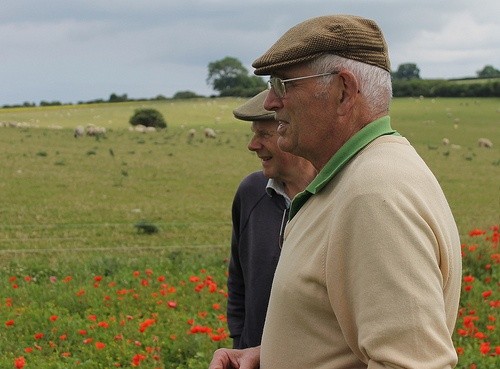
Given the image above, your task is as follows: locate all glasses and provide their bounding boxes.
[267,70,361,98]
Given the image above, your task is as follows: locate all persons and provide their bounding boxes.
[224,85,322,352]
[208,13,463,369]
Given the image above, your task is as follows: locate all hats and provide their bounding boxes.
[252,15,390,76]
[232,89,277,121]
